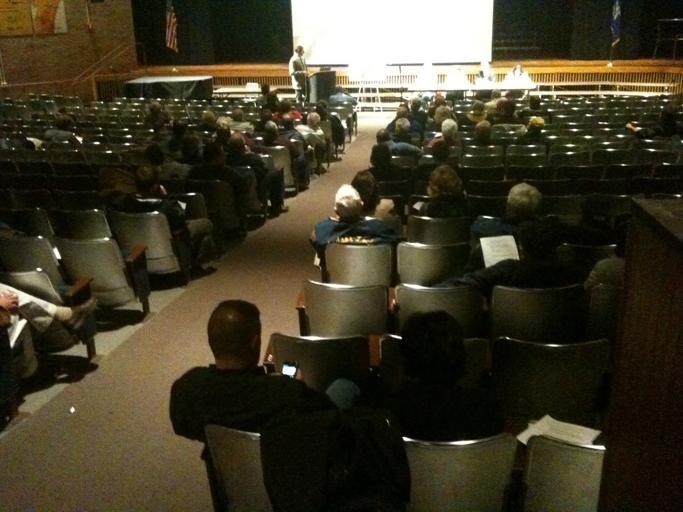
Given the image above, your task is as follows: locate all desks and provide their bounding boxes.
[125,76,213,99]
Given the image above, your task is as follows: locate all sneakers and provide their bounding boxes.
[62,296,96,334]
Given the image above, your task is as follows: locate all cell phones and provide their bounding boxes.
[281,360,299,379]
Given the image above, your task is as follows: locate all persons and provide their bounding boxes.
[0,46,683,511]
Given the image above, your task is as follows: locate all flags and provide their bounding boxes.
[609,0,621,49]
[164,2,180,53]
